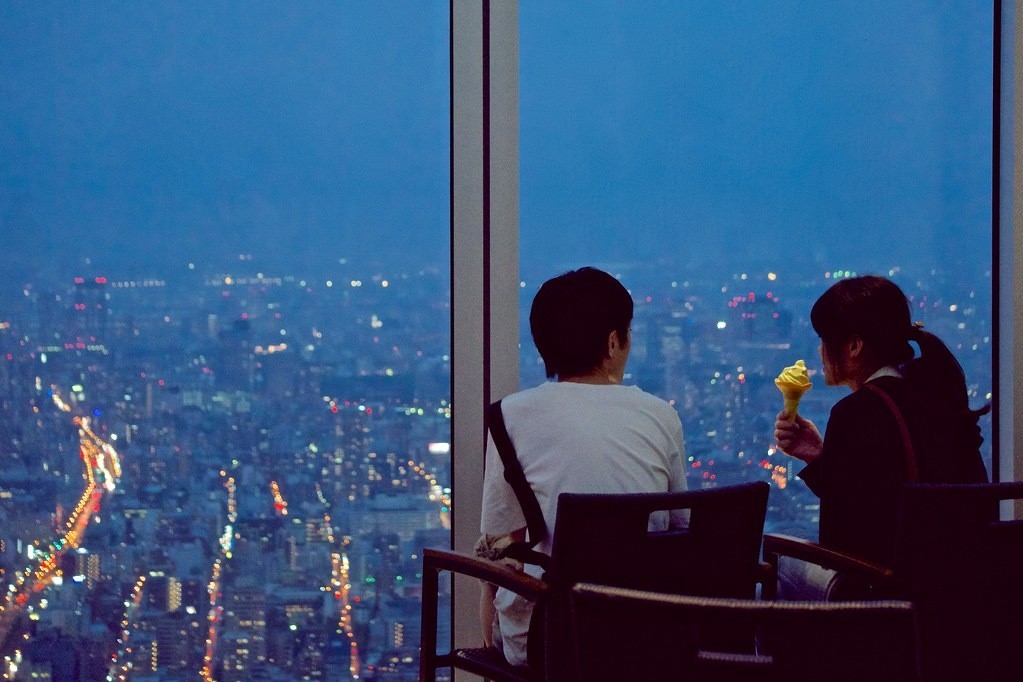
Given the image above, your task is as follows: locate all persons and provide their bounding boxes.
[479,267,693,668]
[756,276,999,612]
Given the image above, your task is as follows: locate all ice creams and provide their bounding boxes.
[774,360,813,423]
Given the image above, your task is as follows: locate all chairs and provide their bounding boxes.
[763,481,1022,682]
[567,584,923,682]
[420,481,770,682]
